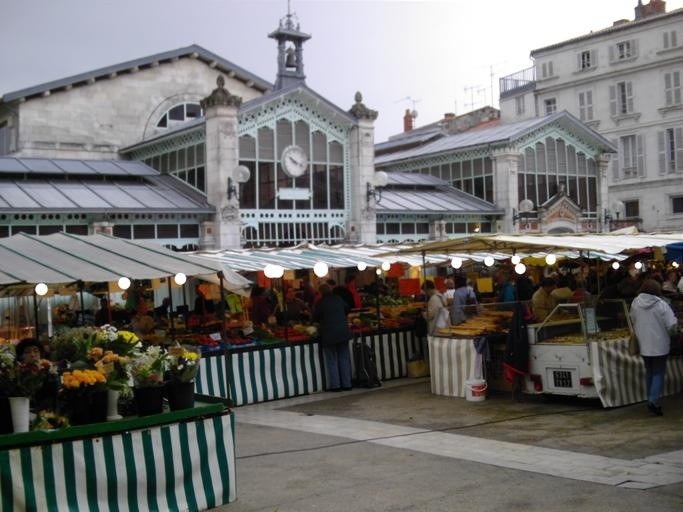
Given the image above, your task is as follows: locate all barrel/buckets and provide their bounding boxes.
[465,379,487,401]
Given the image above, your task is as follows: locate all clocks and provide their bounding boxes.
[282,146,309,177]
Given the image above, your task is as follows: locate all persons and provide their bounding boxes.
[276,286,313,326]
[93,297,119,326]
[416,279,448,341]
[311,283,353,394]
[662,271,679,293]
[567,268,583,288]
[529,275,558,323]
[13,336,50,366]
[151,296,170,321]
[628,279,679,416]
[191,284,217,313]
[451,274,481,325]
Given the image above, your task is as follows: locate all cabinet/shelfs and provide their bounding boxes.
[1,391,237,508]
[429,318,683,405]
[173,321,431,406]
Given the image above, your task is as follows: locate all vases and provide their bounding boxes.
[9,396,31,434]
[166,382,195,412]
[107,389,123,420]
[133,385,166,417]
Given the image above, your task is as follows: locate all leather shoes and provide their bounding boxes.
[647,401,664,417]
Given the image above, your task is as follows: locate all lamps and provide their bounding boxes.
[365,171,388,205]
[513,264,526,276]
[263,265,283,279]
[33,283,48,296]
[511,255,520,264]
[545,253,556,265]
[512,199,538,225]
[314,262,328,278]
[484,256,494,267]
[174,273,186,287]
[118,277,131,291]
[381,261,390,273]
[377,268,381,275]
[603,201,625,225]
[226,165,250,203]
[612,262,620,270]
[358,262,366,271]
[451,256,463,269]
[634,261,643,269]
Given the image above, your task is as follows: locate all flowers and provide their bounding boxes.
[35,411,67,429]
[0,346,60,395]
[163,347,200,384]
[132,344,165,385]
[48,324,142,389]
